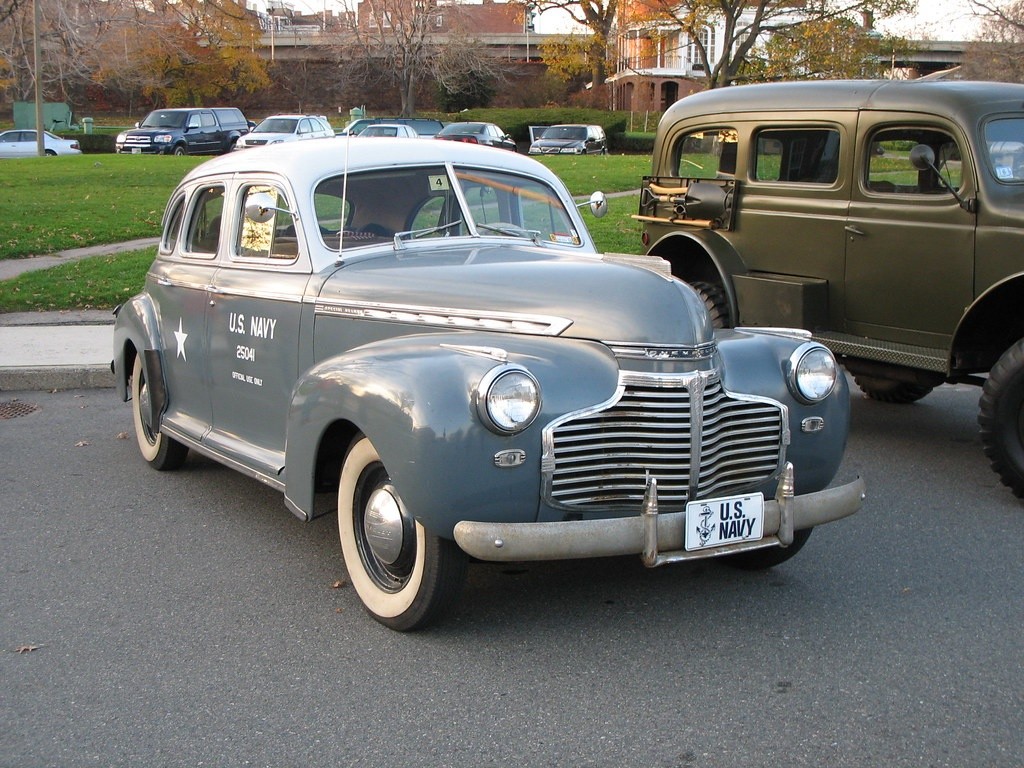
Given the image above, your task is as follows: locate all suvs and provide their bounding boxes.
[337,117,445,138]
[234,114,334,152]
[632,79,1024,495]
[113,107,251,157]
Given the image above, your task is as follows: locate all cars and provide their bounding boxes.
[0,130,83,159]
[435,121,522,153]
[529,124,608,157]
[356,124,417,138]
[108,135,865,630]
